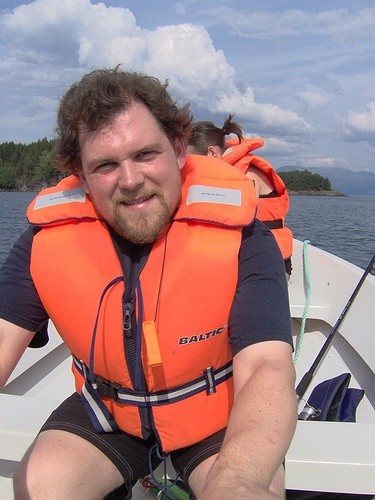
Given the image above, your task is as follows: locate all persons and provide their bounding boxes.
[0,66,296,500]
[184,121,293,284]
